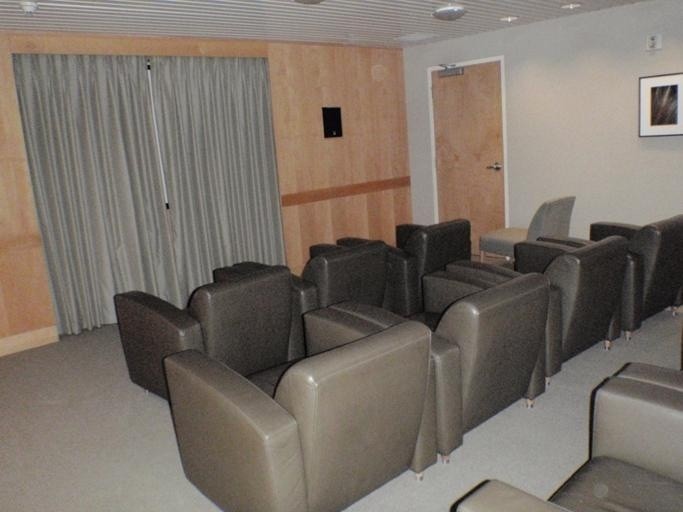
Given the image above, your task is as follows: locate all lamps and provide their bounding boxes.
[432,5,465,21]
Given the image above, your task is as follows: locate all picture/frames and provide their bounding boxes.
[637,72,682,137]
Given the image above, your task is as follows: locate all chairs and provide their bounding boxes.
[477,196,576,264]
[454,375,683,512]
[328,267,551,456]
[447,235,630,374]
[113,265,301,400]
[536,213,682,342]
[163,306,439,512]
[617,361,682,391]
[231,240,387,361]
[336,219,472,312]
